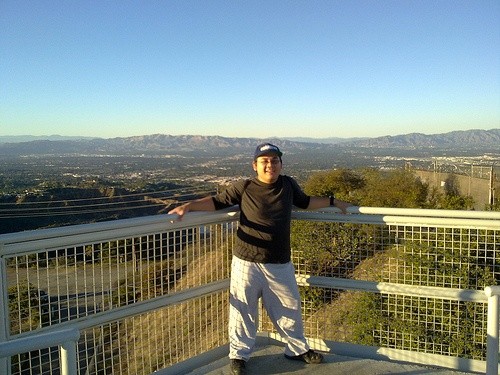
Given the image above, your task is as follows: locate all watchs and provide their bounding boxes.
[329,195,335,207]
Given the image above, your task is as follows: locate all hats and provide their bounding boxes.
[255,143,282,160]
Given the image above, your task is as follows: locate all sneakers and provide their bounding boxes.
[284,349,322,363]
[230,359,247,375]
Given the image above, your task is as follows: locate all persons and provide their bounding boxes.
[167,142,354,375]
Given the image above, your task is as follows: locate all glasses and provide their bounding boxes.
[257,160,281,164]
